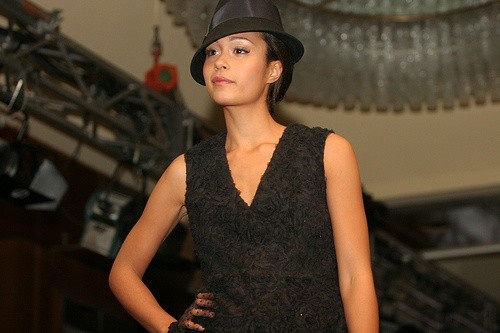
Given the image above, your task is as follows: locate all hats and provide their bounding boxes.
[189,0,304,86]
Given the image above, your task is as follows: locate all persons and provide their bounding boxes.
[108,0,380,333]
